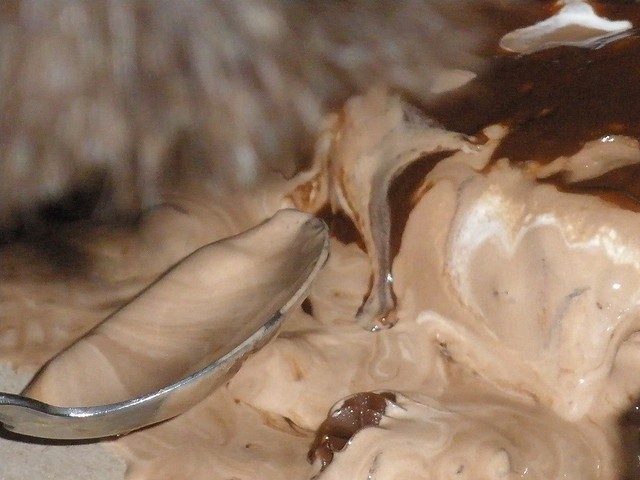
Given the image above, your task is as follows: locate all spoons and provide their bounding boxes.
[0,209,332,441]
